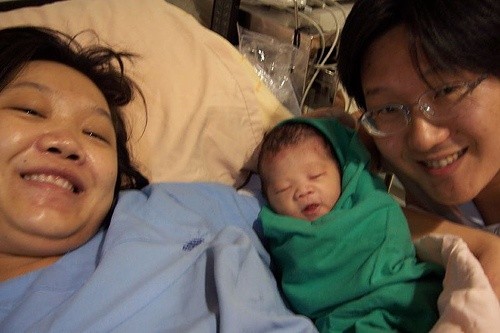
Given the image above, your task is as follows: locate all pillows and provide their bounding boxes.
[0,0,296,194]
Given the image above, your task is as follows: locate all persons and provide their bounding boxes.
[250,113,445,333]
[0,23,321,333]
[337,1,500,237]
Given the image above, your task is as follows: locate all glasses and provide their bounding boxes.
[357,71,496,145]
